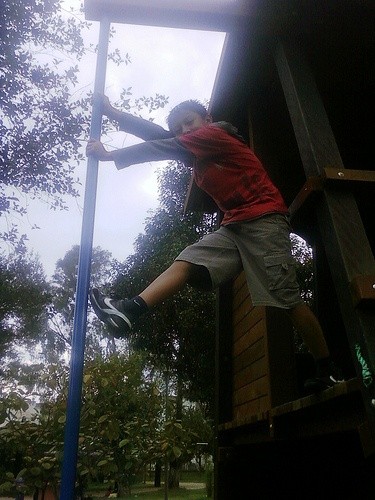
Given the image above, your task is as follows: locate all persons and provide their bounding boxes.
[86,94,346,388]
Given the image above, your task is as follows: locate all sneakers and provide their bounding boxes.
[89,288,135,339]
[304,369,341,392]
